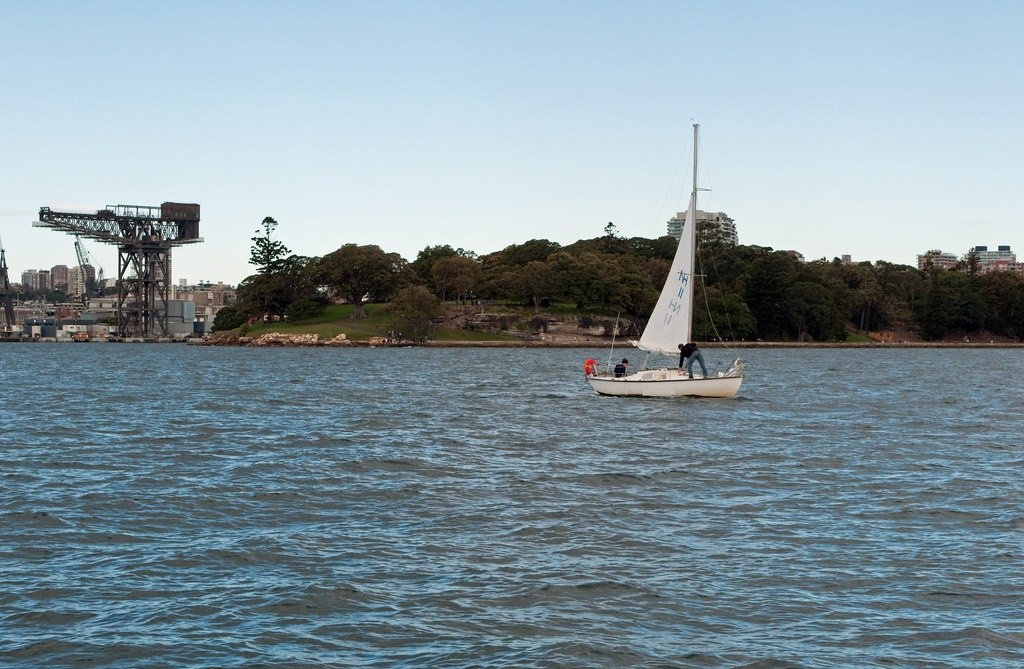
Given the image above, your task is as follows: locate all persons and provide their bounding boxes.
[614,358,628,378]
[385,328,429,345]
[678,343,708,379]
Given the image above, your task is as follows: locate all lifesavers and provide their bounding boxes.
[585,359,596,375]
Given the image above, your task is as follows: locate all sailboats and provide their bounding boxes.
[584,121,746,399]
